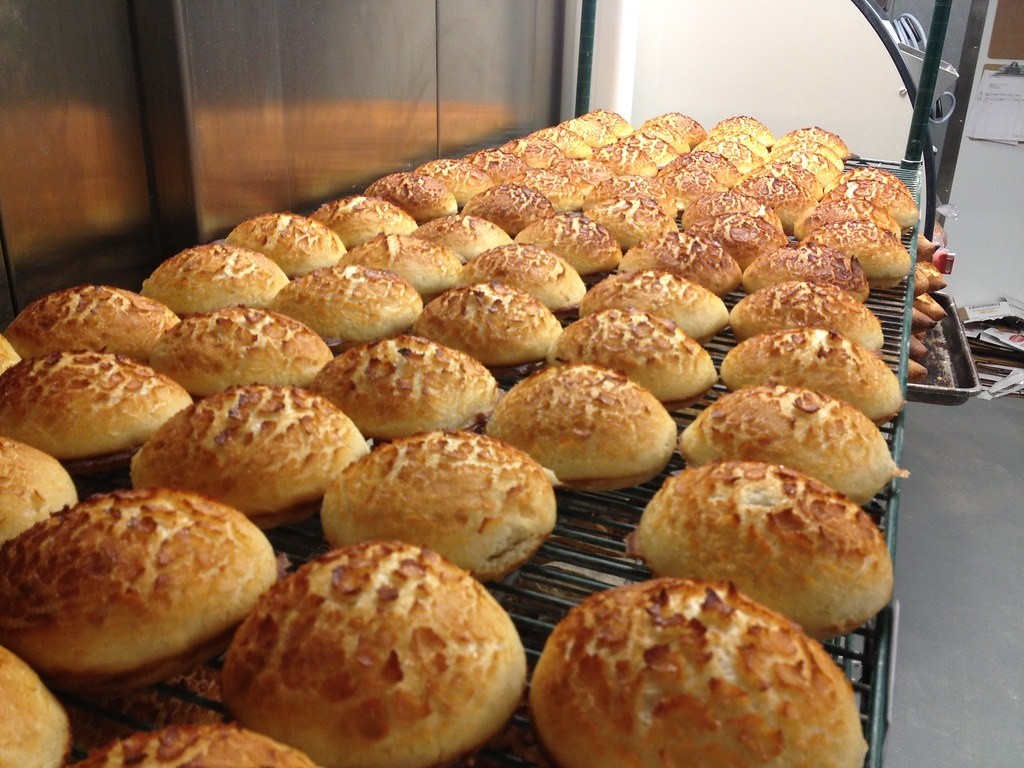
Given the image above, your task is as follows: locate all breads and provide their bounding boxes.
[0,110,956,768]
